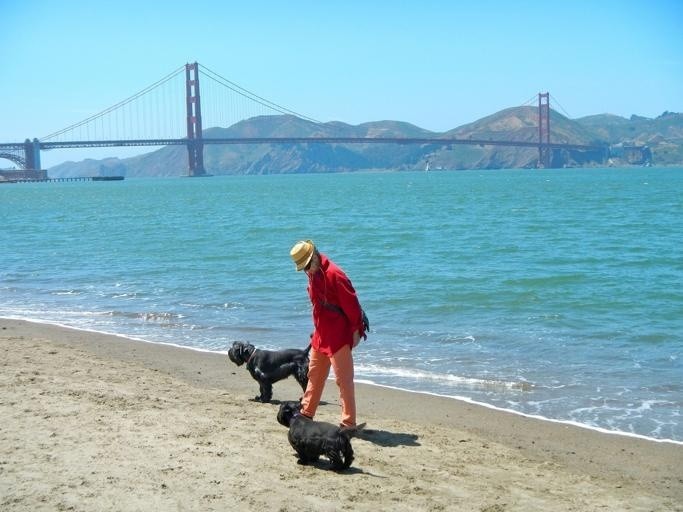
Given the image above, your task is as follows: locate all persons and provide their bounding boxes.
[289,238,367,436]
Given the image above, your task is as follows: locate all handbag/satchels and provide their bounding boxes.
[360,309,369,340]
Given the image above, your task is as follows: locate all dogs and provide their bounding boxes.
[227,333,314,403]
[276,401,367,472]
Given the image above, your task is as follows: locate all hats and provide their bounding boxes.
[291,240,314,270]
